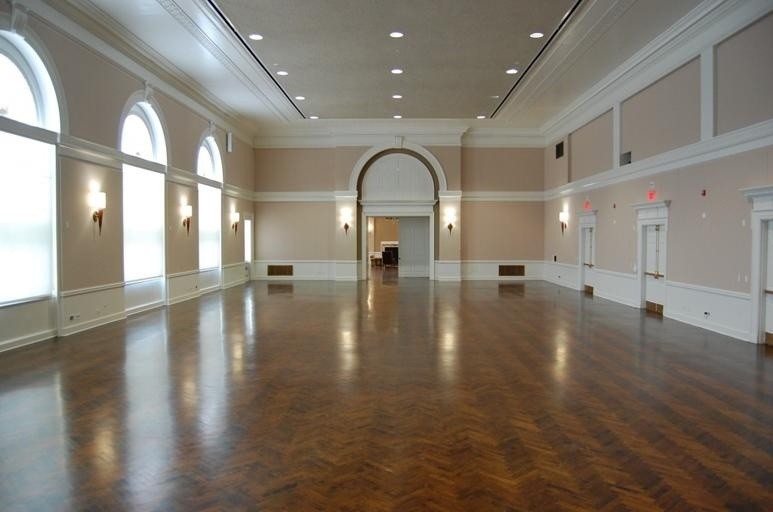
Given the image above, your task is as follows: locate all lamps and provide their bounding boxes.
[183,206,192,229]
[93,192,106,228]
[559,212,567,229]
[232,213,239,229]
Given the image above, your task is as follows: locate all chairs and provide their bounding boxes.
[382,251,396,271]
